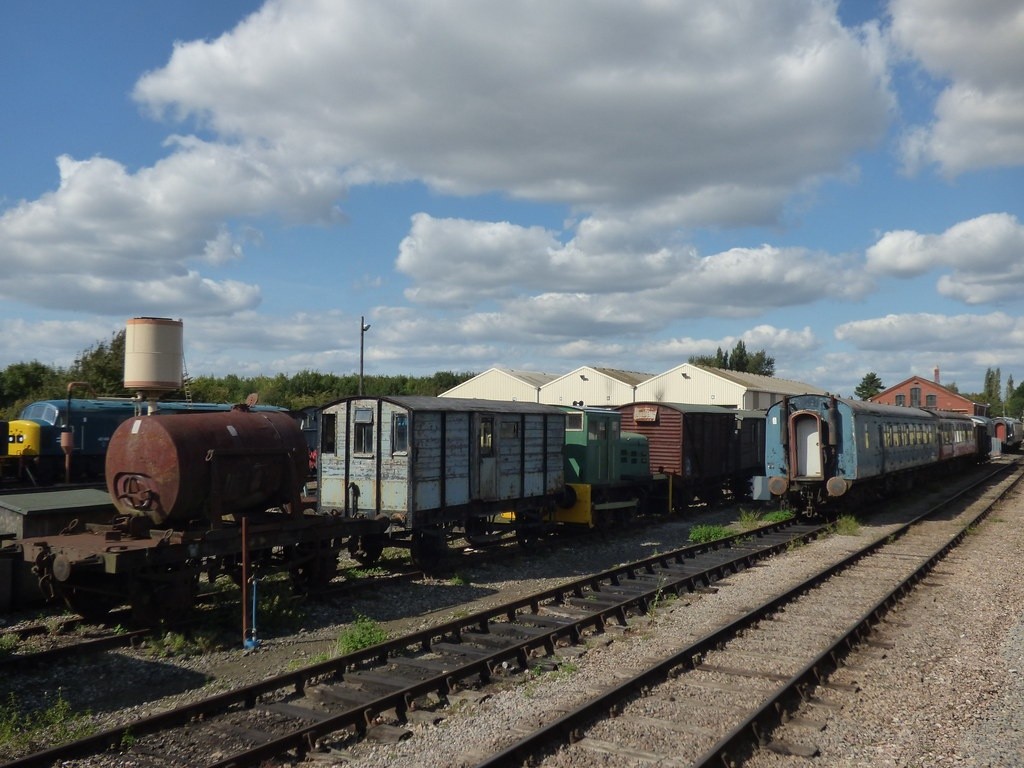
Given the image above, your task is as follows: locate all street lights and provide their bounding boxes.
[359,315,371,397]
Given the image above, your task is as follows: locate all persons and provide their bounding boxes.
[308,447,317,471]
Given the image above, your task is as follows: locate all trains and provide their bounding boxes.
[1,395,768,620]
[765,392,1024,519]
